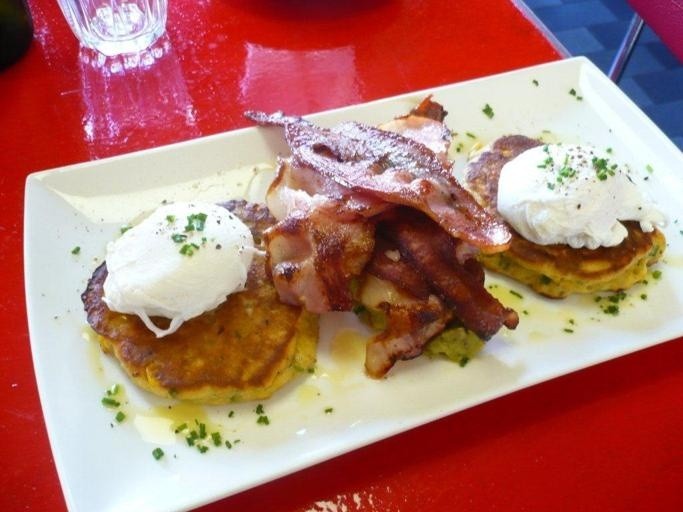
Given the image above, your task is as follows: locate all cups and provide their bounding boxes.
[77,34,203,163]
[54,0,169,57]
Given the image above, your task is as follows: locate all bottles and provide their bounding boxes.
[0,0,34,71]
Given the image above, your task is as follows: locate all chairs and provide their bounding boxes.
[608,0,683,85]
